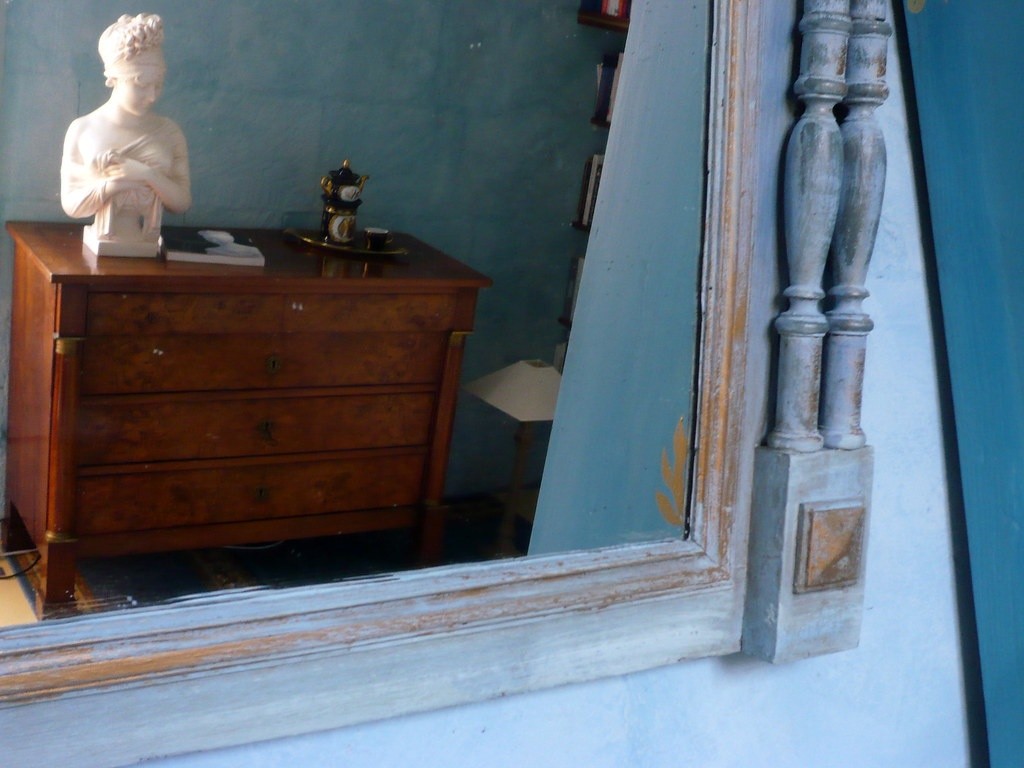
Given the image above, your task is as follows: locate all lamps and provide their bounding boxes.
[465,353,564,559]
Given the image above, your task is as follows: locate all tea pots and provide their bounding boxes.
[321,159,370,202]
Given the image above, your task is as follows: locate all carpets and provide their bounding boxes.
[7,498,540,620]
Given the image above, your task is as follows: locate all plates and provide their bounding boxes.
[304,230,409,254]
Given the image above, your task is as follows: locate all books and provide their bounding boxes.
[577,155,605,227]
[596,51,624,123]
[161,231,264,267]
[580,0,628,19]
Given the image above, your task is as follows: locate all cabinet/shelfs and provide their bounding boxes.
[560,12,633,332]
[0,219,495,620]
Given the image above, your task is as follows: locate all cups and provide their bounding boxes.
[364,226,394,250]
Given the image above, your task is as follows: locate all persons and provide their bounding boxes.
[60,12,193,242]
